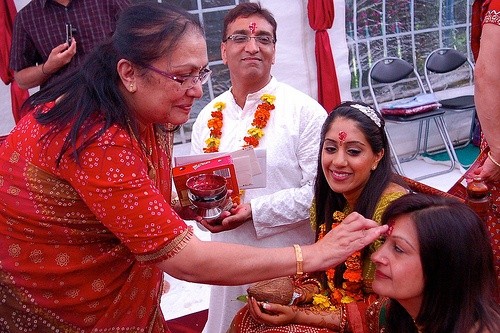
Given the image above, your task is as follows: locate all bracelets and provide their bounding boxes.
[488,151,500,167]
[41,64,50,76]
[294,244,303,278]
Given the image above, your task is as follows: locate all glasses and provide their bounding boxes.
[223,34,277,46]
[145,64,213,90]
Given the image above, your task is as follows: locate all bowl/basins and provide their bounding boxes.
[185,173,227,198]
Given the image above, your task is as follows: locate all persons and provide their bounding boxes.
[369,194,500,333]
[469,0,487,147]
[226,100,408,333]
[9,0,158,100]
[0,1,389,333]
[190,1,330,333]
[466,0,500,314]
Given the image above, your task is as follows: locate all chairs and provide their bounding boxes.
[368,56,456,182]
[421,48,484,175]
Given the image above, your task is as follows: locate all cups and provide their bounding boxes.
[187,190,227,218]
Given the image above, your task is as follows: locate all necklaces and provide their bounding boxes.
[202,93,276,153]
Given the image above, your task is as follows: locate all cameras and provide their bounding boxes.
[65,23,77,48]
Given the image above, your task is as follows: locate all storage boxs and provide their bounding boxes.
[172,155,240,208]
